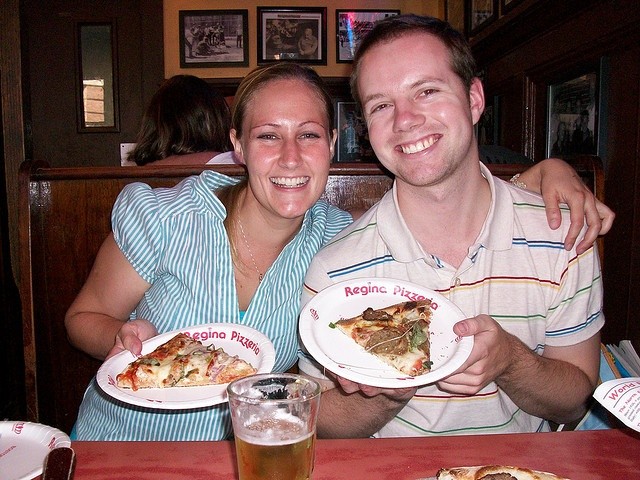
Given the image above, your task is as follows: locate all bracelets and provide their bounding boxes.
[509,172,527,191]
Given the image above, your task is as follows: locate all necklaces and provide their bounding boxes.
[236,186,265,283]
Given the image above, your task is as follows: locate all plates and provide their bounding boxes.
[95,321,277,411]
[298,277,476,390]
[0,417,74,480]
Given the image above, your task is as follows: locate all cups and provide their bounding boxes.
[223,371,322,480]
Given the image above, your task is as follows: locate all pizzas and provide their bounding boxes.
[335,298,435,377]
[116,332,258,392]
[438,466,560,480]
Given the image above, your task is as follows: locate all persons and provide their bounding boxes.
[185,22,232,59]
[340,35,344,47]
[267,20,318,59]
[236,24,243,47]
[283,14,605,439]
[126,76,232,165]
[548,109,595,159]
[64,61,616,442]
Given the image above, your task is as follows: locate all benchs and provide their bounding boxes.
[11,161,620,434]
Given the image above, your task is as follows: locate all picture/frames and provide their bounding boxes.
[178,8,250,68]
[256,5,328,65]
[524,74,609,162]
[335,7,402,63]
[331,93,384,163]
